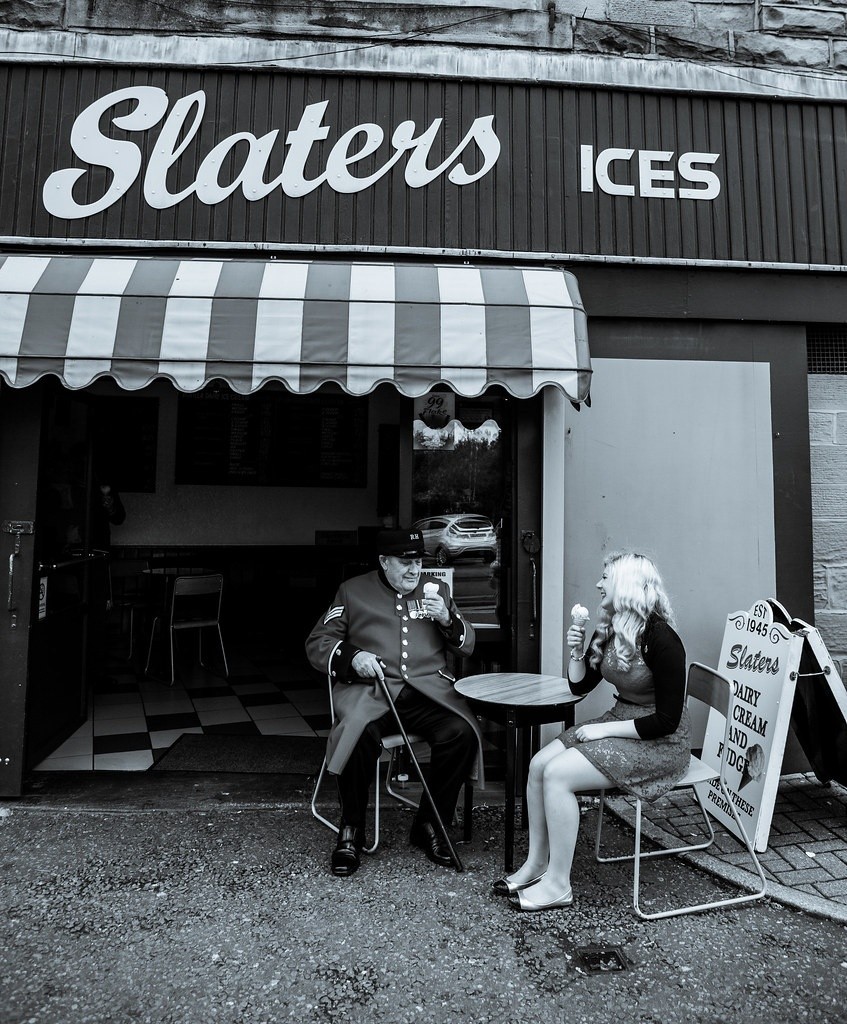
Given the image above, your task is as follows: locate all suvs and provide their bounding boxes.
[412,513,497,567]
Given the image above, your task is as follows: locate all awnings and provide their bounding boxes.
[0,248,594,413]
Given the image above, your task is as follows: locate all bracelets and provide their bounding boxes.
[570,649,585,662]
[353,650,363,658]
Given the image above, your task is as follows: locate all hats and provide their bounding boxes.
[379,530,433,558]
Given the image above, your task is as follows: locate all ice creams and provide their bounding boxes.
[100,485,111,496]
[571,603,590,632]
[423,582,439,621]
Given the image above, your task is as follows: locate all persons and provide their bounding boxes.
[62,442,126,547]
[493,548,691,910]
[305,528,486,878]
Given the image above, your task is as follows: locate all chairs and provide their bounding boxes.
[594,661,768,920]
[312,675,457,854]
[144,574,229,686]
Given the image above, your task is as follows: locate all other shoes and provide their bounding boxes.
[491,871,548,894]
[506,887,573,910]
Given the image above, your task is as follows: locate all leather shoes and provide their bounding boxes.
[332,823,366,876]
[409,819,455,868]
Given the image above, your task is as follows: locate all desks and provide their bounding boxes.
[453,672,590,872]
